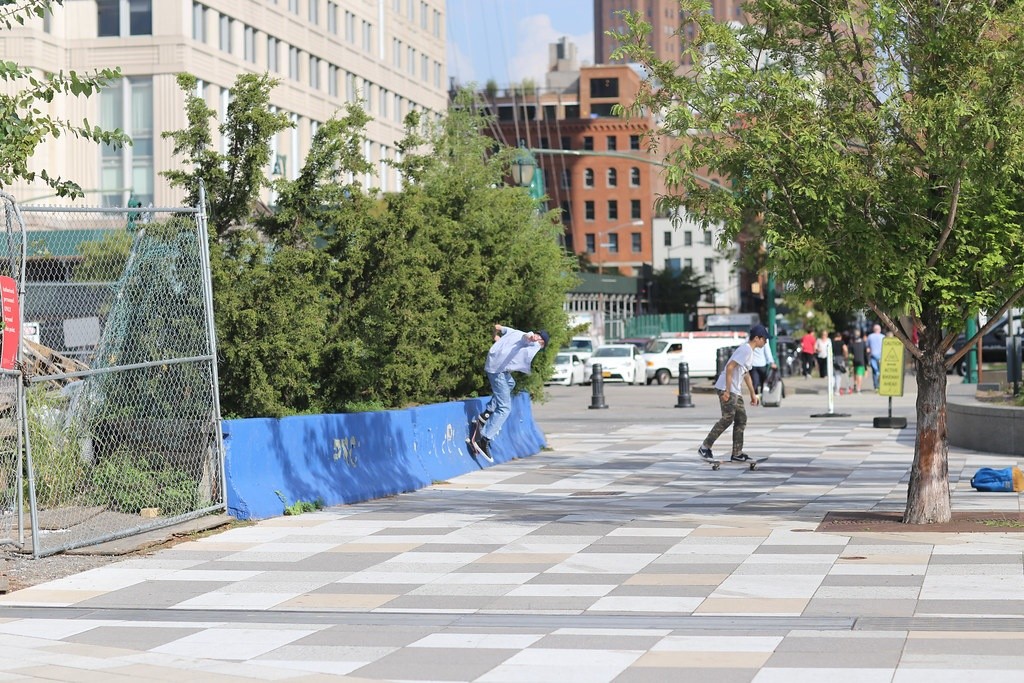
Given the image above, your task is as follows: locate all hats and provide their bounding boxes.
[750,325,773,339]
[536,330,548,348]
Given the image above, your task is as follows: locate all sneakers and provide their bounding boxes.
[731,452,756,463]
[478,409,491,424]
[698,445,714,462]
[472,437,494,463]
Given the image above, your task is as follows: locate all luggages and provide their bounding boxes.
[761,365,782,406]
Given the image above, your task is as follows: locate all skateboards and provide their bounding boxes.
[699,454,769,471]
[465,414,482,455]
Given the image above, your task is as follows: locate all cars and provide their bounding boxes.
[585,344,649,386]
[542,352,584,386]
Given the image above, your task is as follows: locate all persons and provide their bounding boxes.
[732,324,899,407]
[697,324,775,464]
[465,323,551,462]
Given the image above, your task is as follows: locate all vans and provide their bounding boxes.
[641,337,748,387]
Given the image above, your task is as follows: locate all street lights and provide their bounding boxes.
[510,139,538,187]
